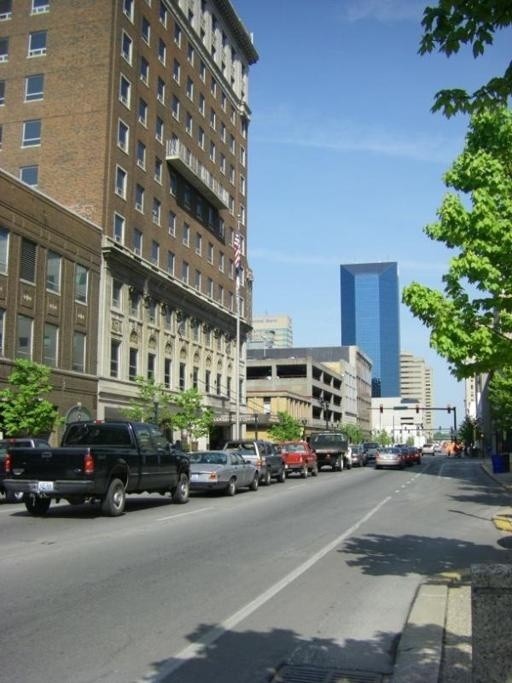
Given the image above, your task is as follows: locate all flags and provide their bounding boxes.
[233,220,242,268]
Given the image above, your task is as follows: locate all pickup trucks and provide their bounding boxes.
[0,436,52,502]
[3,419,192,517]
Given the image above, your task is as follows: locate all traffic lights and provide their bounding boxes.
[416,404,419,412]
[448,405,451,413]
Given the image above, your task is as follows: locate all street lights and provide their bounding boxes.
[253,409,259,440]
[480,433,485,457]
[380,403,384,412]
[151,392,160,418]
[301,416,308,440]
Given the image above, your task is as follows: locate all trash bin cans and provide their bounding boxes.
[492,454,510,473]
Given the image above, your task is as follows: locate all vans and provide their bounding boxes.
[222,439,286,484]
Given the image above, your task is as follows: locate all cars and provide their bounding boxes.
[185,450,261,494]
[275,440,320,477]
[349,439,443,470]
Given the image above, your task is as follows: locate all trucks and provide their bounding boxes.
[309,429,353,471]
